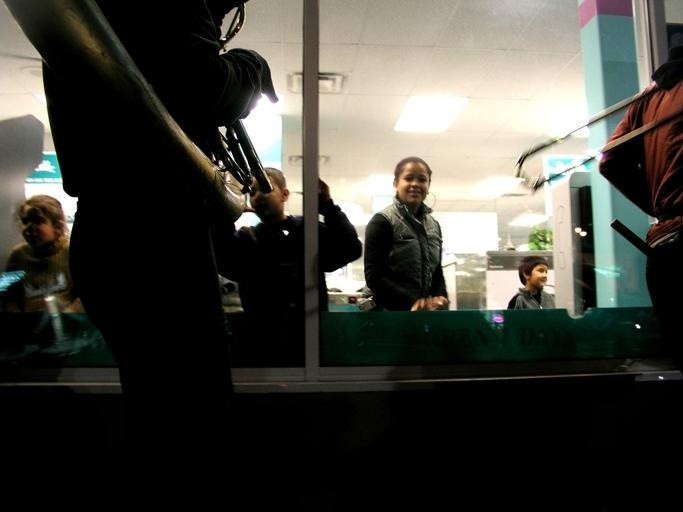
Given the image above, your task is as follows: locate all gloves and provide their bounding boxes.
[235,49,278,103]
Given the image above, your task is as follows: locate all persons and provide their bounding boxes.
[362,157,452,312]
[211,166,363,313]
[597,43,682,382]
[39,2,279,512]
[1,193,88,315]
[507,254,557,311]
